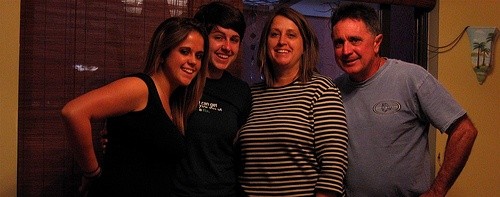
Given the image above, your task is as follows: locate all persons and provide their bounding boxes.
[231,6,348,197]
[99,1,253,196]
[323,2,478,197]
[60,16,210,197]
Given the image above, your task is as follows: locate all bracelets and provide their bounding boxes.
[80,163,103,180]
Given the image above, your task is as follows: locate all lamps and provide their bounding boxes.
[466,26,500,85]
